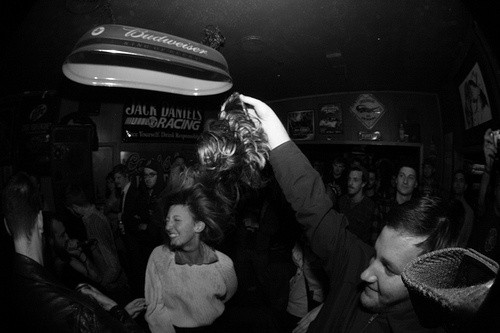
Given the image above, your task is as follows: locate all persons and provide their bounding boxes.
[239,92,468,333]
[144,192,238,333]
[0,129,500,333]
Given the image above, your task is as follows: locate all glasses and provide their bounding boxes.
[143,173,159,178]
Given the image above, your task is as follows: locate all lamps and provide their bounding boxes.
[60,24,234,97]
